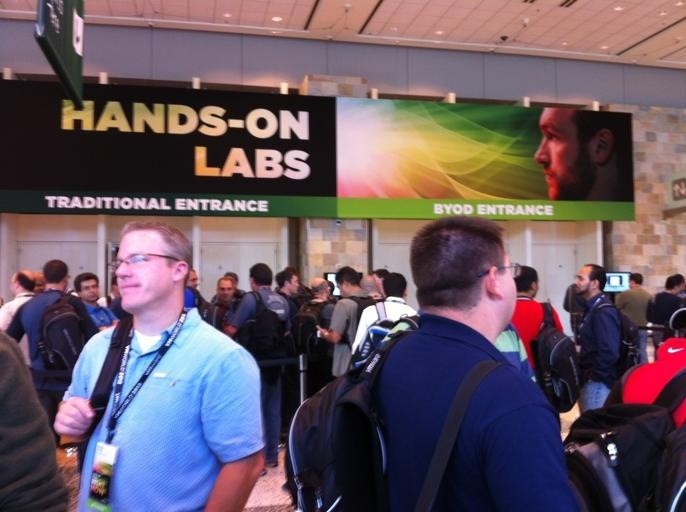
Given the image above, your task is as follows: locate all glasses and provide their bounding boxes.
[478,264,523,278]
[107,253,181,272]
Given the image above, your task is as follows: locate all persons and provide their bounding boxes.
[534,108,631,200]
[602,307,686,511]
[52,219,266,510]
[1,260,129,471]
[183,262,418,466]
[493,263,686,427]
[353,216,590,509]
[0,329,73,511]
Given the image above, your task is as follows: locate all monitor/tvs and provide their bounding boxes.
[324,273,362,297]
[603,272,631,293]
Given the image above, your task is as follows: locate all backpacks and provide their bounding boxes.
[562,362,686,512]
[528,302,584,412]
[236,291,283,357]
[36,294,90,371]
[292,300,336,353]
[594,302,641,378]
[283,329,390,512]
[351,302,395,369]
